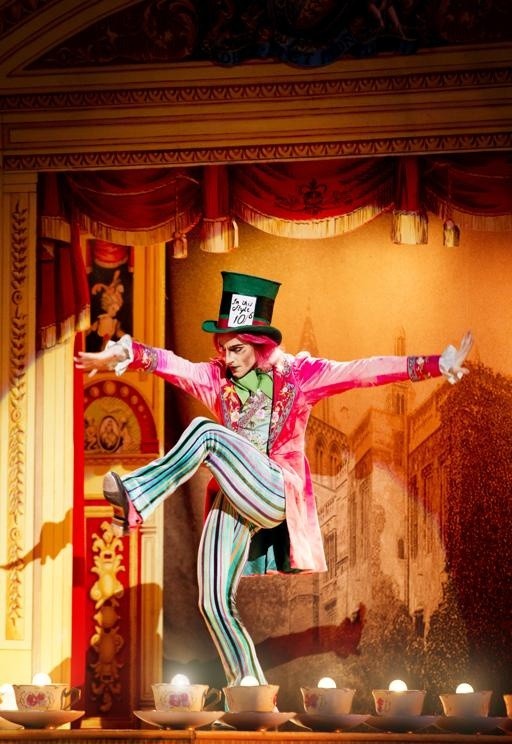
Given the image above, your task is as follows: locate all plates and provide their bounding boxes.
[290,714,373,735]
[1,710,86,730]
[435,716,502,734]
[133,710,226,731]
[218,712,297,731]
[365,715,438,733]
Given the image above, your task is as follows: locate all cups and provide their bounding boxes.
[9,682,80,710]
[150,682,221,711]
[298,685,356,710]
[436,689,492,710]
[370,686,427,708]
[221,685,279,710]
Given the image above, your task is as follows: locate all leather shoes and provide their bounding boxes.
[102,470,143,539]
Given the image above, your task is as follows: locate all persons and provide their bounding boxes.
[74,333,474,730]
[87,292,125,352]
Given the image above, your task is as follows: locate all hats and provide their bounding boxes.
[201,270,283,347]
